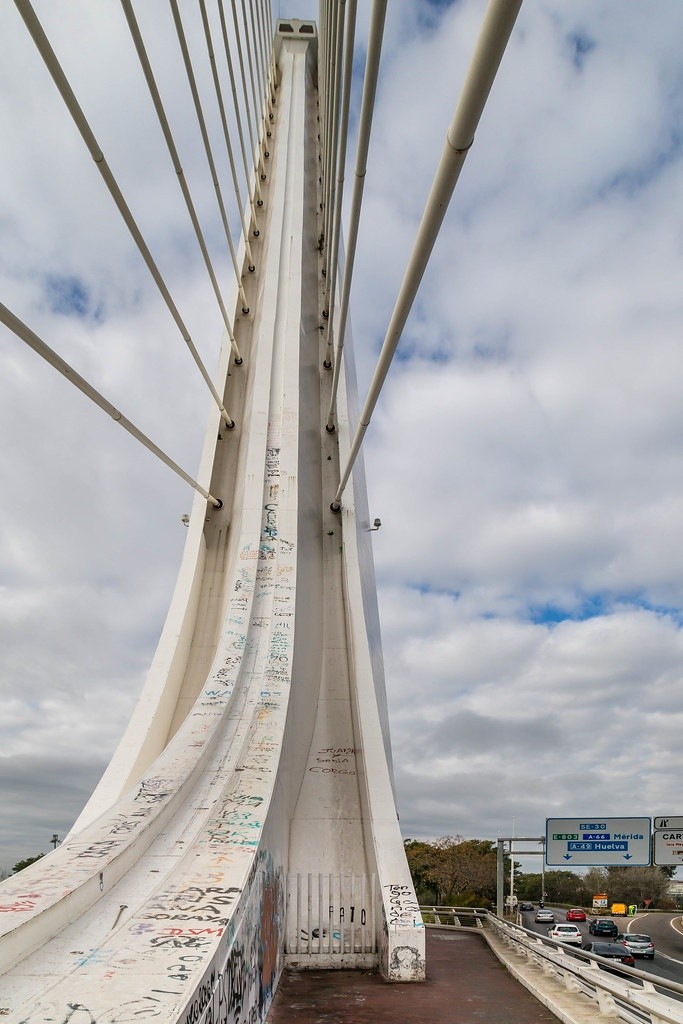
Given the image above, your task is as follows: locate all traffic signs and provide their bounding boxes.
[545,816,652,867]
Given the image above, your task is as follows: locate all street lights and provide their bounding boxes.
[509,815,516,916]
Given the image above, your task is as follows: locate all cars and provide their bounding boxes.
[519,902,535,912]
[534,909,555,924]
[574,941,636,978]
[546,923,583,947]
[565,908,587,922]
[611,933,655,960]
[588,918,618,937]
[610,902,628,917]
